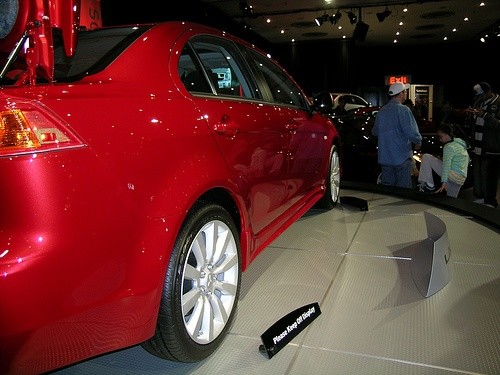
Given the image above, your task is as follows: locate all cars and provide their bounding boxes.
[346,105,473,185]
[309,91,373,114]
[0,19,344,375]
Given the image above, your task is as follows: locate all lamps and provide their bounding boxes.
[329,9,342,25]
[315,10,329,27]
[347,8,356,24]
[376,4,391,22]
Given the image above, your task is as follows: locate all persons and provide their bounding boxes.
[402,98,418,127]
[333,97,347,113]
[466,82,500,208]
[415,123,471,198]
[421,97,428,120]
[372,81,422,188]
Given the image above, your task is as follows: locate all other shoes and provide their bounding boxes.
[416,182,435,192]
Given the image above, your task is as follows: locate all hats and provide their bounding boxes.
[474,82,491,96]
[388,81,411,96]
